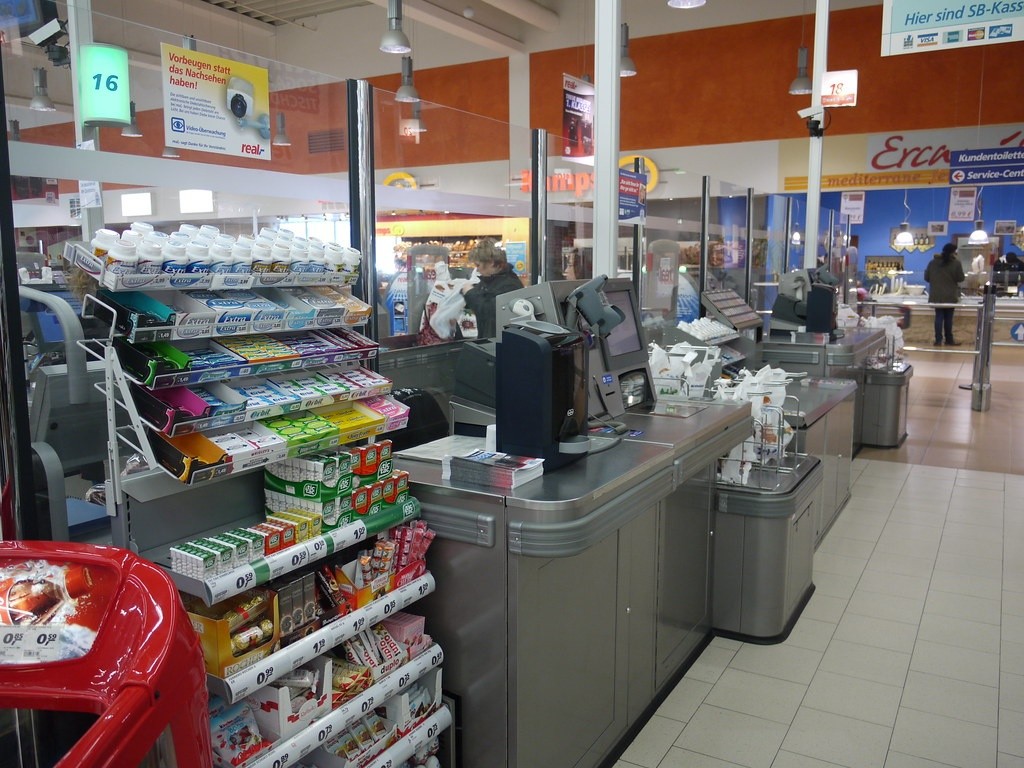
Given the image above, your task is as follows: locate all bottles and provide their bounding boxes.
[1,566,93,625]
[91,221,362,274]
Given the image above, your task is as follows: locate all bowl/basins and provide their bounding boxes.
[905,285,926,295]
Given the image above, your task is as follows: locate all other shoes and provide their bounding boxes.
[945,339,961,346]
[934,341,941,346]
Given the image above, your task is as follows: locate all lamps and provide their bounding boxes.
[379,0,411,54]
[396,57,421,103]
[894,188,914,246]
[793,199,800,241]
[619,22,638,78]
[789,48,813,94]
[968,187,988,244]
[410,103,427,132]
[7,69,291,159]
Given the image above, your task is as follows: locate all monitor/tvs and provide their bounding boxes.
[0,0,59,43]
[605,290,643,356]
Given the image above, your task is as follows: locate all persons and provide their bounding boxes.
[454,236,525,341]
[564,246,592,279]
[925,243,965,345]
[994,251,1024,287]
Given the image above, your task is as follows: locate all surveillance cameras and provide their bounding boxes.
[226,75,255,118]
[797,105,823,119]
[27,18,66,47]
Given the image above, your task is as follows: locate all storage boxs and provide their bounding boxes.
[60,239,442,768]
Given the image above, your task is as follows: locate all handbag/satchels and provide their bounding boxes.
[415,279,472,344]
